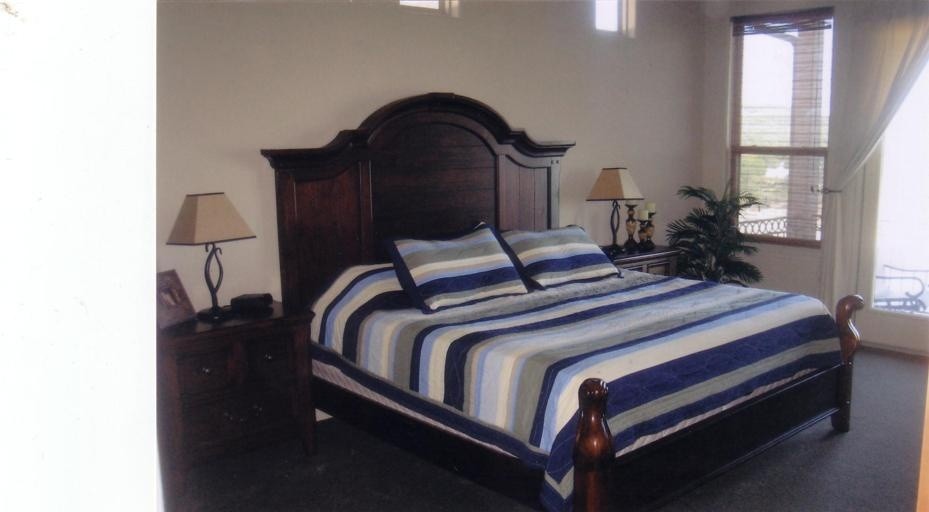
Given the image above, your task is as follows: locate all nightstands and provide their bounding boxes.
[157,299,318,496]
[599,245,682,276]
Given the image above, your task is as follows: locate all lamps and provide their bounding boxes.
[165,192,256,320]
[585,168,645,257]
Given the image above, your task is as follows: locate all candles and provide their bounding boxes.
[638,209,649,220]
[625,200,637,205]
[645,203,655,213]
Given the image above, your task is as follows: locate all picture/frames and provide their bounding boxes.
[156,270,198,332]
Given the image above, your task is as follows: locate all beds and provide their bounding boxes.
[262,92,865,512]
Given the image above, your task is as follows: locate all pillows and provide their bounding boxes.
[498,225,624,290]
[382,219,534,312]
